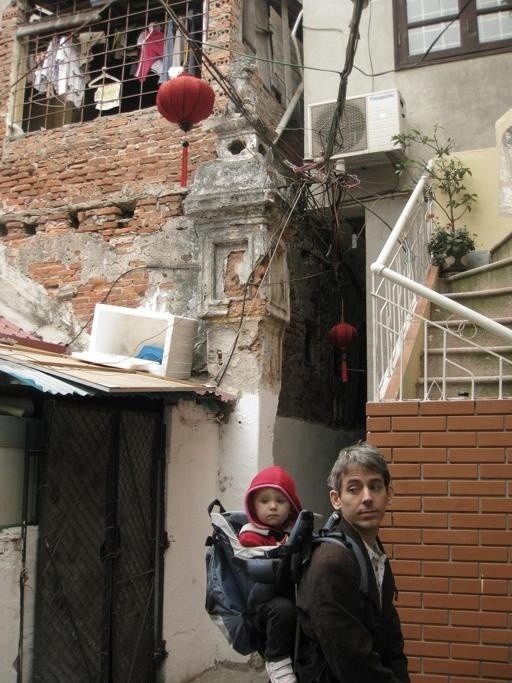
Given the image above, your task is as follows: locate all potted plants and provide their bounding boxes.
[387,123,480,276]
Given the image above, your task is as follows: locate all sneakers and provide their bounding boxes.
[265,656,297,683]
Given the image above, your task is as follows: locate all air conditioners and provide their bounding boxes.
[306,88,407,174]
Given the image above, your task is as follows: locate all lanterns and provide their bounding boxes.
[156,72,215,187]
[326,319,359,384]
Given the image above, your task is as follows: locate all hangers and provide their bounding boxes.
[88,62,120,86]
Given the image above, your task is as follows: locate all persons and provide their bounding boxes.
[296,443,411,682]
[238,465,302,682]
